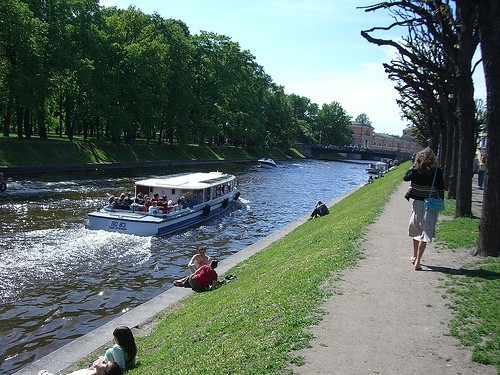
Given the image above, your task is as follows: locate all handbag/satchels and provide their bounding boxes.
[425,198,446,211]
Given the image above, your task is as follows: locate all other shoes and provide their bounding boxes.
[308,218,314,220]
[315,216,318,217]
[415,266,422,270]
[413,260,415,264]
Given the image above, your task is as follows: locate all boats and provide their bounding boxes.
[257,157,277,169]
[85,170,240,237]
[366,157,399,174]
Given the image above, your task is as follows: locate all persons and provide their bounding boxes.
[38,326,137,375]
[367,176,373,184]
[478,156,486,189]
[403,147,445,270]
[180,189,220,206]
[381,171,383,177]
[188,246,211,273]
[173,260,218,292]
[109,192,167,214]
[308,201,327,220]
